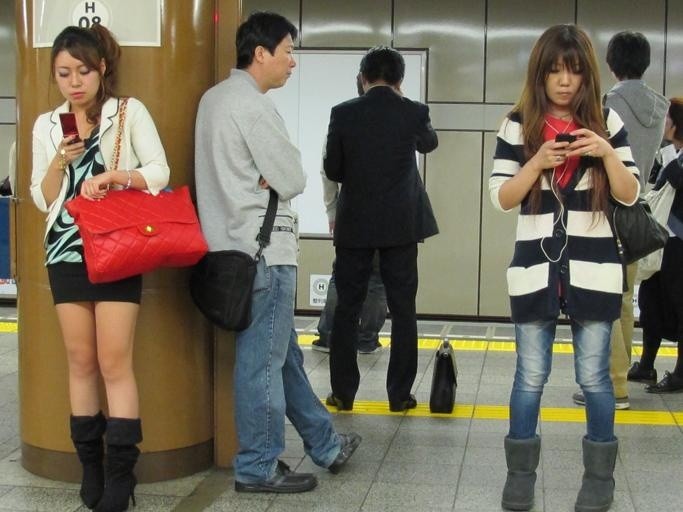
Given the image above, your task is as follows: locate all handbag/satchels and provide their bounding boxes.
[603,185,671,263]
[429,337,459,413]
[63,94,211,291]
[635,161,679,284]
[182,241,258,334]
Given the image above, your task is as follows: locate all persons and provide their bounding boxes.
[572,29,673,410]
[28,23,172,511]
[487,22,644,512]
[312,72,389,354]
[322,42,441,411]
[627,95,683,394]
[192,8,362,493]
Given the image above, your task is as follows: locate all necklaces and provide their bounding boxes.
[546,109,571,120]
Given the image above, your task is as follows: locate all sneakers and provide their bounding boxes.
[233,459,318,494]
[359,338,384,354]
[311,339,331,353]
[572,388,631,410]
[327,431,362,476]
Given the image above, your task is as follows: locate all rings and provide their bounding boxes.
[556,155,561,161]
[584,151,591,157]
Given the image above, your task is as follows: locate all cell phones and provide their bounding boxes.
[555,132,579,144]
[58,112,82,144]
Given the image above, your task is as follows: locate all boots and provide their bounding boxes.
[573,435,619,512]
[68,410,108,507]
[100,412,142,512]
[501,431,542,512]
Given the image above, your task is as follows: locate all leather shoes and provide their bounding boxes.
[628,360,658,384]
[387,392,417,413]
[326,391,355,411]
[644,369,683,394]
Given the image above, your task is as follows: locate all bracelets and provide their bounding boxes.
[124,168,133,190]
